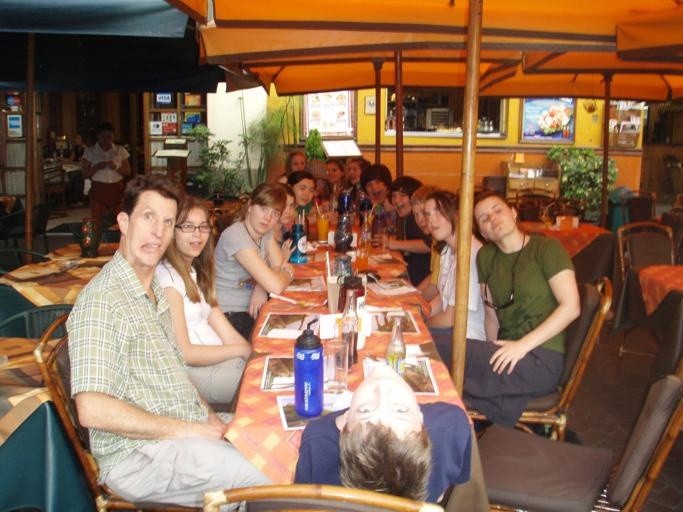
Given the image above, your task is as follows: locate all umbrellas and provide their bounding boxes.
[1,0,225,264]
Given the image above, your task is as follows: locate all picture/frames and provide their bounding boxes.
[298,86,358,145]
[517,92,579,146]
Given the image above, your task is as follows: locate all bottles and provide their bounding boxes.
[335,272,366,310]
[340,288,358,366]
[288,223,308,263]
[293,318,325,418]
[385,314,408,377]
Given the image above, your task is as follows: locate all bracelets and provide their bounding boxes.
[93,166,97,170]
[113,165,119,171]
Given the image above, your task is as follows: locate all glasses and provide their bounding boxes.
[484,271,515,309]
[176,225,214,233]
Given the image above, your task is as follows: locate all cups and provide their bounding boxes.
[315,182,388,271]
[322,338,350,391]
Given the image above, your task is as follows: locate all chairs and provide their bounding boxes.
[476,364,683,512]
[30,313,205,511]
[465,275,612,442]
[0,169,118,341]
[202,482,449,512]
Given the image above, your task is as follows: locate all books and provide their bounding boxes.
[1,91,76,196]
[149,91,206,183]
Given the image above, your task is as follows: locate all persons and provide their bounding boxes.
[295,369,471,511]
[72,133,88,159]
[64,174,273,510]
[82,122,131,217]
[210,150,583,430]
[149,196,253,405]
